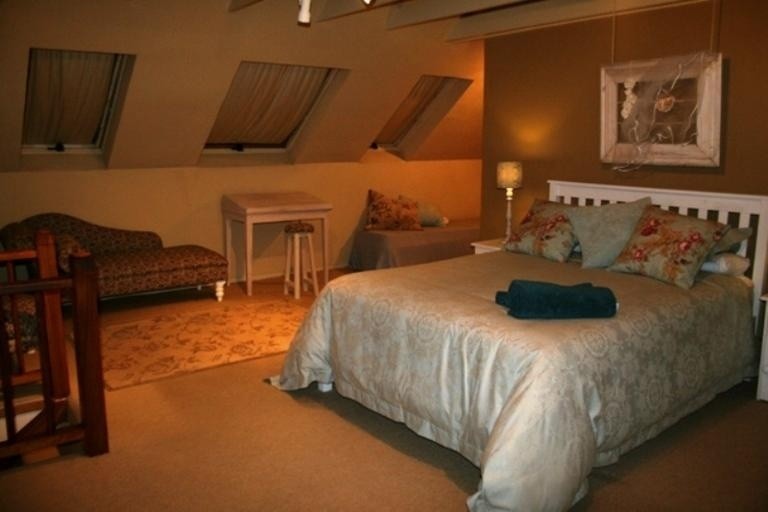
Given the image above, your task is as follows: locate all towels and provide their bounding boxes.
[494,281,592,307]
[507,277,615,322]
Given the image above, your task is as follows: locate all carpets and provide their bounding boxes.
[66,296,313,393]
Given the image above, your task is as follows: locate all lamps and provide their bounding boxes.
[494,159,524,246]
[296,0,318,26]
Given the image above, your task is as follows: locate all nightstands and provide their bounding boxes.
[470,236,511,256]
[751,295,768,402]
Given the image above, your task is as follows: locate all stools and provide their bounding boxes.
[279,219,320,300]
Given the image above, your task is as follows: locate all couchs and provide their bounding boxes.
[1,213,229,365]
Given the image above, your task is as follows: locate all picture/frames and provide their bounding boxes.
[597,55,722,168]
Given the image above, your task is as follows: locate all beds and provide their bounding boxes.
[260,177,767,511]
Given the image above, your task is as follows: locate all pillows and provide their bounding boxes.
[402,194,448,228]
[705,220,752,261]
[611,203,724,296]
[566,195,654,269]
[363,189,419,233]
[33,230,89,272]
[699,250,750,277]
[501,193,590,265]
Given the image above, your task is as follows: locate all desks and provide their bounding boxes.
[220,192,332,301]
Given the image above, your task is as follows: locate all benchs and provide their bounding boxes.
[349,219,479,276]
